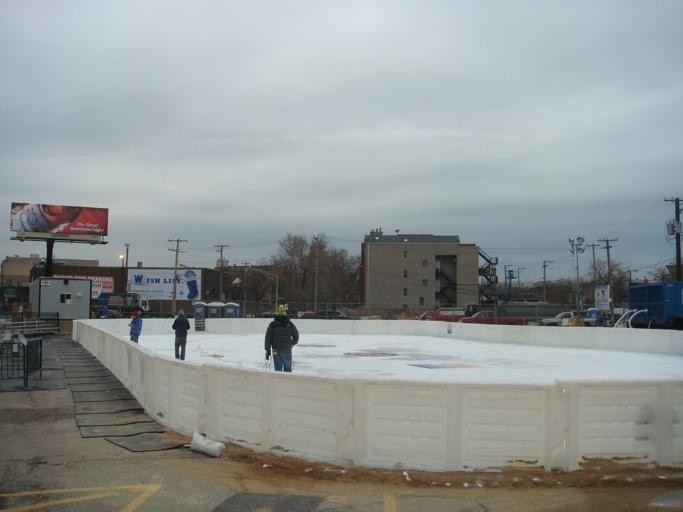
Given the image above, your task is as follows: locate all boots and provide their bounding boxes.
[180,355,185,360]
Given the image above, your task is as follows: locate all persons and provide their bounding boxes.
[128,309,142,343]
[172,309,190,360]
[265,304,299,372]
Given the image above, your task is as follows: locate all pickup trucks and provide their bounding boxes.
[540,312,596,327]
[410,308,525,326]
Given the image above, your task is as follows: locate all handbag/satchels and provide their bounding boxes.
[272,348,280,356]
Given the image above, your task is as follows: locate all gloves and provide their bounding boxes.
[128,322,135,328]
[265,350,271,360]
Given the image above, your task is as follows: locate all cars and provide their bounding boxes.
[302,309,360,320]
[258,308,298,318]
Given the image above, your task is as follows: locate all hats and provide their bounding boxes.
[179,309,184,315]
[277,305,287,315]
[131,310,140,315]
